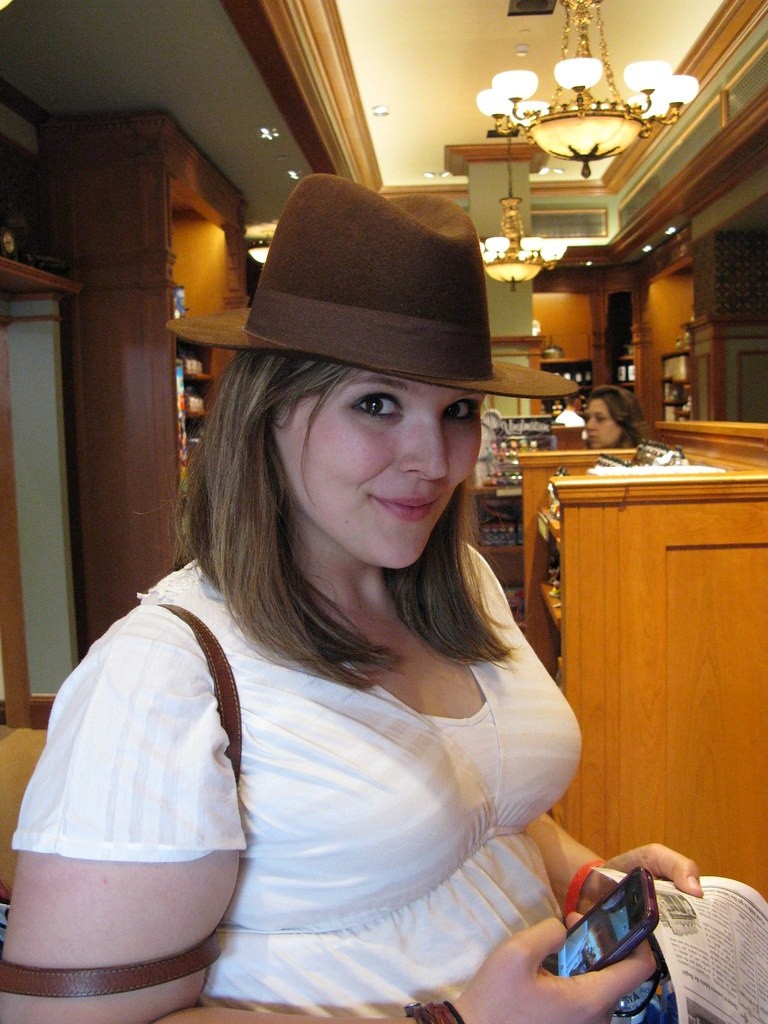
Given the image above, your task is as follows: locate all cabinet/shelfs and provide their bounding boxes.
[169,340,221,465]
[475,354,634,481]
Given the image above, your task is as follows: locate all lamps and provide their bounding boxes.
[474,127,570,291]
[473,0,704,180]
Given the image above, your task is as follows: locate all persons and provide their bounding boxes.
[0,173,707,1024]
[586,384,650,450]
[552,396,589,447]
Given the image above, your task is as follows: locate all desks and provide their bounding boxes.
[474,486,524,546]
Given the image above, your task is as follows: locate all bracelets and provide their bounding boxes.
[404,1001,466,1024]
[562,858,605,918]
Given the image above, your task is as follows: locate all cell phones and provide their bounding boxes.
[540,866,659,979]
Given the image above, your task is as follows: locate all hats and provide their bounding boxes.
[165,172,579,400]
